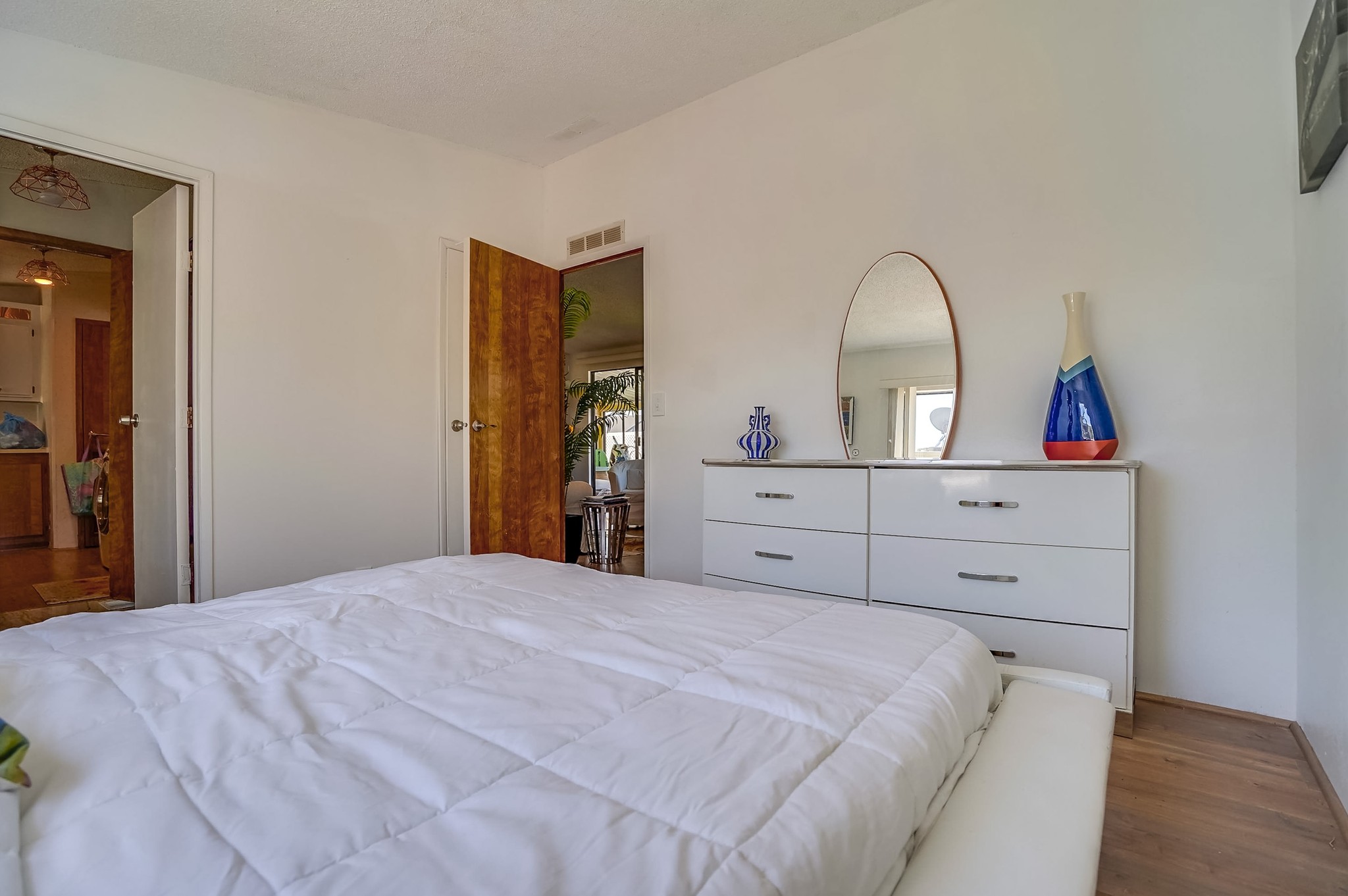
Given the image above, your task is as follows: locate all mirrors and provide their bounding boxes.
[837,252,959,458]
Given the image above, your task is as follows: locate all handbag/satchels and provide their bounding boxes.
[61,432,104,516]
[0,412,45,448]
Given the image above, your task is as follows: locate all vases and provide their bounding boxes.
[737,406,781,460]
[1042,292,1119,459]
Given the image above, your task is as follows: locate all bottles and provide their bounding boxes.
[736,407,780,459]
[1041,290,1120,462]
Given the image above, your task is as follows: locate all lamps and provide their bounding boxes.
[15,245,71,286]
[9,145,91,210]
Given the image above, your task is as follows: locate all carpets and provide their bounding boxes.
[31,573,110,605]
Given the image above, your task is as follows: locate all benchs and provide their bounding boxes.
[892,663,1114,896]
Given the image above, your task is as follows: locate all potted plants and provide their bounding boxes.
[559,288,644,564]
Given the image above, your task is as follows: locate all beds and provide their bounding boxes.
[0,551,1003,896]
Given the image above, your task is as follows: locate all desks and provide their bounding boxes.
[579,501,631,564]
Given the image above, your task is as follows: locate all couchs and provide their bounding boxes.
[609,459,644,531]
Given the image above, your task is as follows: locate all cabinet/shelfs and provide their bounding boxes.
[704,464,1140,738]
[0,453,49,549]
[0,318,43,402]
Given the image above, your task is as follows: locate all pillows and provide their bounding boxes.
[626,469,644,489]
